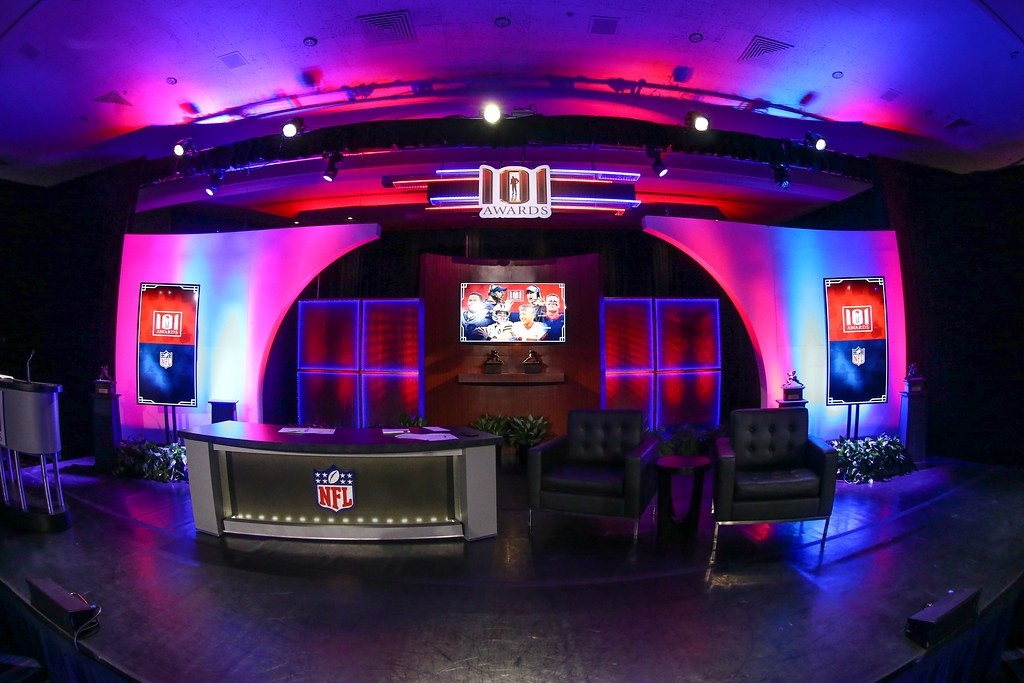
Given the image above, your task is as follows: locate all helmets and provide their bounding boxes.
[492,304,511,322]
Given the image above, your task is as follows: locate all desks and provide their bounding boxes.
[655,453,710,542]
[177,420,502,542]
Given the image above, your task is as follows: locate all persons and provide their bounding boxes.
[462,285,564,341]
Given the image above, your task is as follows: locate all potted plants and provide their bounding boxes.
[506,415,547,468]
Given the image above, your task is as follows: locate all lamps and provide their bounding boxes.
[282,116,303,138]
[323,151,345,183]
[205,168,221,196]
[647,149,669,177]
[684,112,710,134]
[173,137,195,156]
[806,130,826,150]
[772,165,790,189]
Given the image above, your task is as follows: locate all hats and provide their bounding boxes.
[489,285,506,292]
[526,285,539,293]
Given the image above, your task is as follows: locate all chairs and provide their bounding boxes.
[526,409,658,541]
[712,407,839,541]
